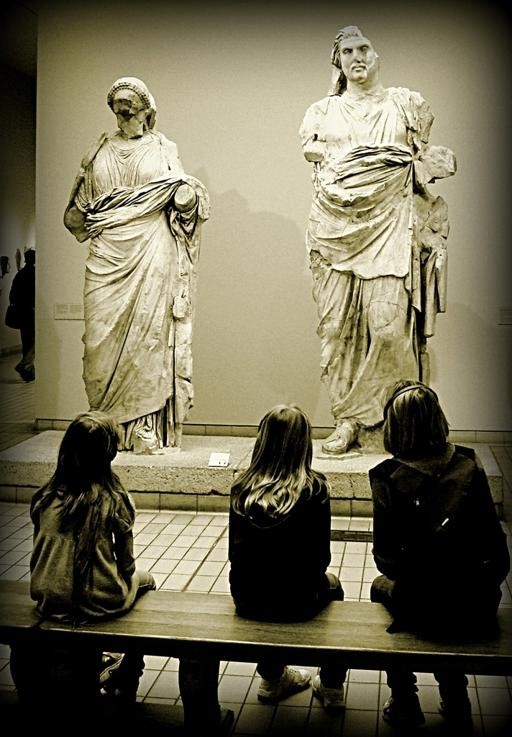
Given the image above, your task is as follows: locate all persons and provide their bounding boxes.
[63,76,212,455]
[300,26,456,455]
[9,250,35,382]
[371,383,510,727]
[30,415,156,688]
[229,409,345,713]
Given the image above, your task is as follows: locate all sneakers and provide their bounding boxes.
[254,666,310,705]
[310,679,347,714]
[98,653,122,675]
[99,670,139,699]
[381,693,428,734]
[437,697,475,730]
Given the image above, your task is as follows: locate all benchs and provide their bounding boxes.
[0,579,512,737]
